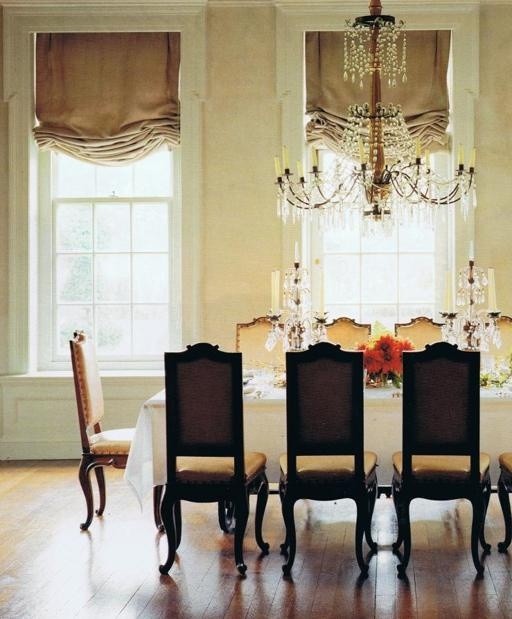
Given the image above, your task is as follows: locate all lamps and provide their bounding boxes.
[273,0,478,237]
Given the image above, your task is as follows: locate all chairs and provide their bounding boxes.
[278,341,379,574]
[158,341,271,574]
[391,341,492,574]
[236,315,303,367]
[495,451,512,554]
[395,317,448,350]
[71,327,165,535]
[315,316,372,351]
[475,315,511,365]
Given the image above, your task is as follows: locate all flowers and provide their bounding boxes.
[356,334,416,388]
